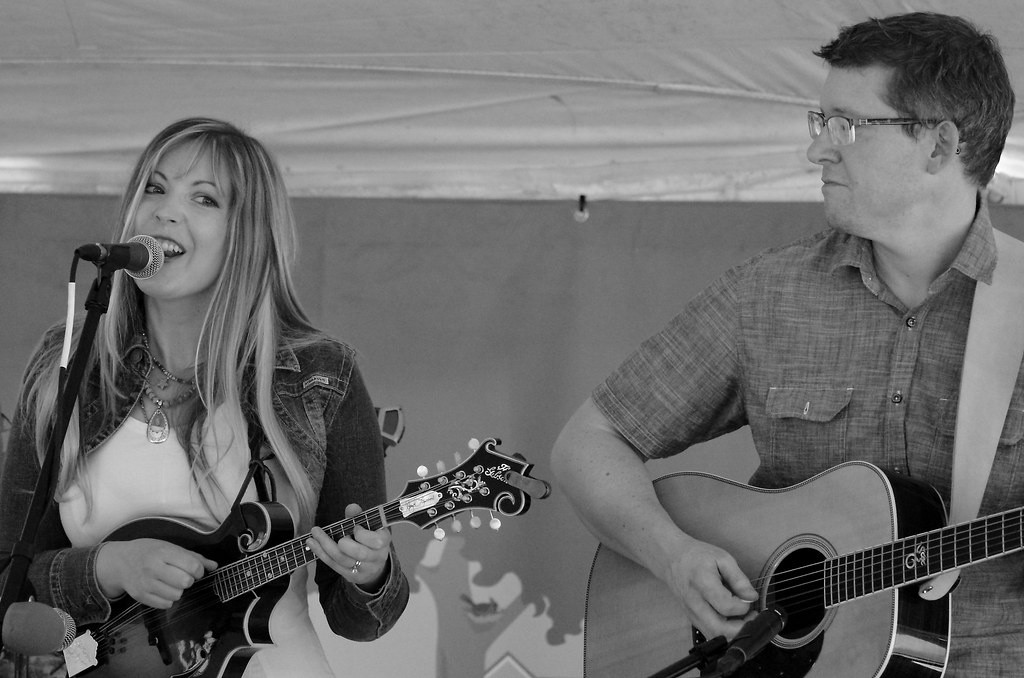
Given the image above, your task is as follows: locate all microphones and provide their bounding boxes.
[0,601,76,655]
[77,235,164,280]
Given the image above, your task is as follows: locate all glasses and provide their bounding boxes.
[807,109,935,146]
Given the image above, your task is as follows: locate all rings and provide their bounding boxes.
[352,561,362,571]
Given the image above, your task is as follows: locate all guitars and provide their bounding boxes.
[577,452,1024,678]
[0,428,557,678]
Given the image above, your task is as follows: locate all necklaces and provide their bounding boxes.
[139,327,204,444]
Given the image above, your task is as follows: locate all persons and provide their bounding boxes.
[0,117,410,678]
[549,12,1024,678]
